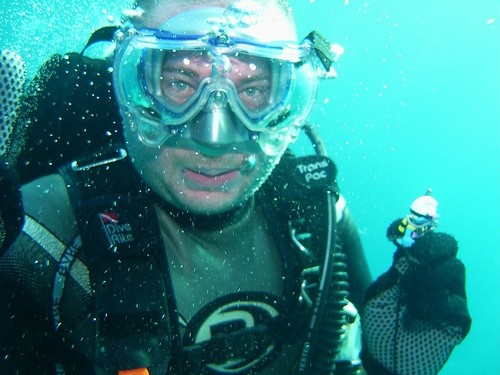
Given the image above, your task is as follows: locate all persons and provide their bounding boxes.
[1,0,472,375]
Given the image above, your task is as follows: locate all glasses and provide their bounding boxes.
[112,25,319,157]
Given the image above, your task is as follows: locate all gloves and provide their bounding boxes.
[0,160,25,259]
[360,218,472,375]
